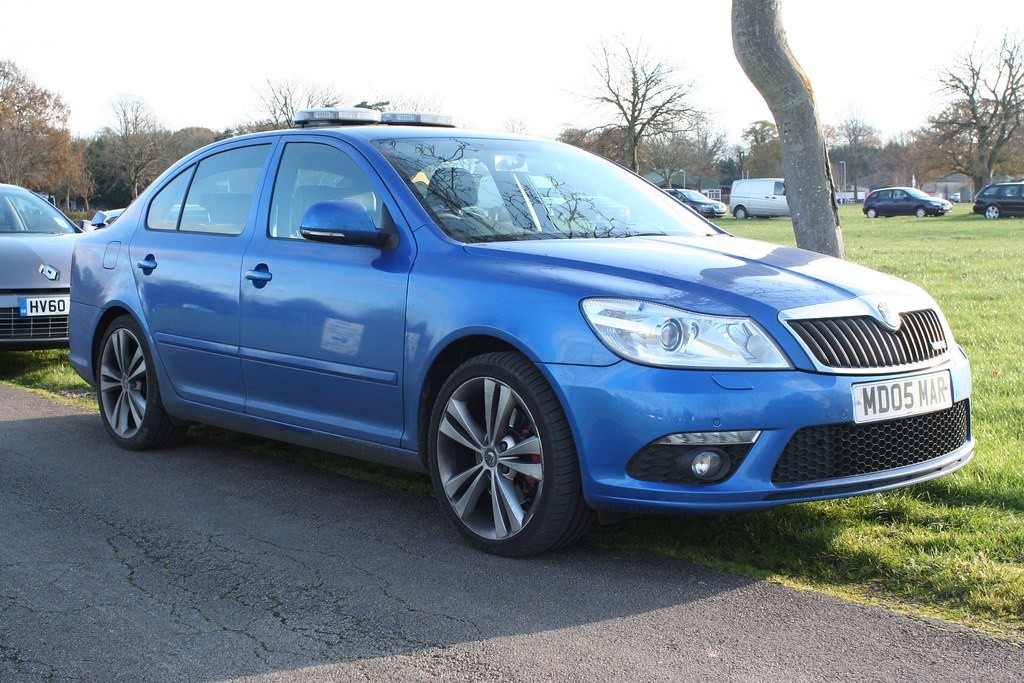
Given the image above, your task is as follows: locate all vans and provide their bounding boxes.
[729,177,793,221]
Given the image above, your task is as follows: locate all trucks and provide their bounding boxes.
[951,192,973,202]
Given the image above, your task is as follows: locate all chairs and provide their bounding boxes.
[293,186,339,203]
[200,191,250,223]
[423,167,479,211]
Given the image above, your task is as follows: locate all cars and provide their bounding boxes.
[973,178,1024,219]
[0,181,88,345]
[862,187,954,219]
[40,193,57,206]
[89,203,212,234]
[61,99,978,564]
[663,188,727,218]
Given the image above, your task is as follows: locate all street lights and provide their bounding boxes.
[679,169,686,189]
[839,160,848,192]
